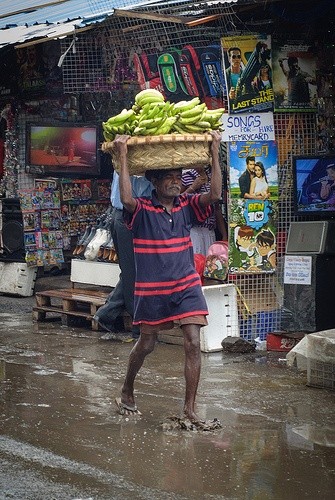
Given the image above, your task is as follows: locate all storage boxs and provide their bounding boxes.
[307,329,335,391]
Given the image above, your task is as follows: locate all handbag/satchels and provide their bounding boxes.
[78,63,133,122]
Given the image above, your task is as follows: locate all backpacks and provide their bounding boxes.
[193,254,206,286]
[133,52,168,102]
[203,241,229,280]
[157,45,229,102]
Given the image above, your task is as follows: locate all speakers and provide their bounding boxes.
[0,198,25,263]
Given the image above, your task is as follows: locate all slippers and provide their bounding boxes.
[180,415,220,431]
[115,397,143,417]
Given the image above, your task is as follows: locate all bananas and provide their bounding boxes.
[101,88,225,142]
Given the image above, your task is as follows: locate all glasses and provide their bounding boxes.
[231,54,242,59]
[261,66,269,69]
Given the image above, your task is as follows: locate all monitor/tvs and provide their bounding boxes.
[292,155,335,217]
[25,121,103,179]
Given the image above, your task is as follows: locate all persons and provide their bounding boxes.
[226,47,317,107]
[317,163,334,200]
[182,165,224,254]
[239,156,271,200]
[93,168,157,340]
[115,131,222,431]
[67,138,76,163]
[318,114,326,150]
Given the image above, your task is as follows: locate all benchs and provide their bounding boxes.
[33,289,113,330]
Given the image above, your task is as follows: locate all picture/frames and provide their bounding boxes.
[293,154,335,215]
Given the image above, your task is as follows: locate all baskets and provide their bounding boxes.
[101,133,222,177]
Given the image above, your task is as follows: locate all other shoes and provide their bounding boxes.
[93,313,119,333]
[132,329,140,338]
[72,226,118,263]
